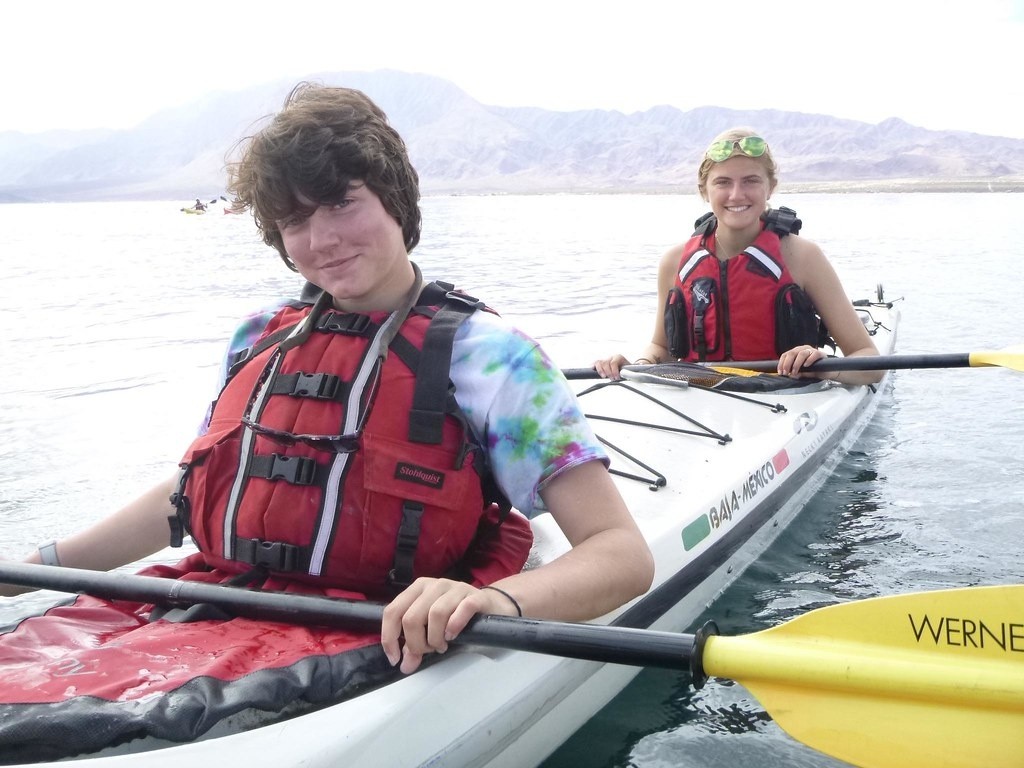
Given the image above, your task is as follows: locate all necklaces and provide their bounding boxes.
[714,231,733,260]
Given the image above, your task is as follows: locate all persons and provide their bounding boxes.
[591,126,886,386]
[0,76,657,681]
[230,197,244,211]
[191,198,209,211]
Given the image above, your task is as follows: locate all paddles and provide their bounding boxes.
[220,196,232,205]
[561,351,1024,380]
[180,199,217,211]
[1,558,1024,768]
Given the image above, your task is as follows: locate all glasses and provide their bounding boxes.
[706,136,768,163]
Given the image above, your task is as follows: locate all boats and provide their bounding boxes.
[223,207,238,216]
[184,209,206,216]
[2,293,897,768]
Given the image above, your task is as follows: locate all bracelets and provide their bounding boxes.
[36,538,62,567]
[482,583,524,619]
[634,357,657,365]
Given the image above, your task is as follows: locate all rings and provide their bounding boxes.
[806,348,812,355]
